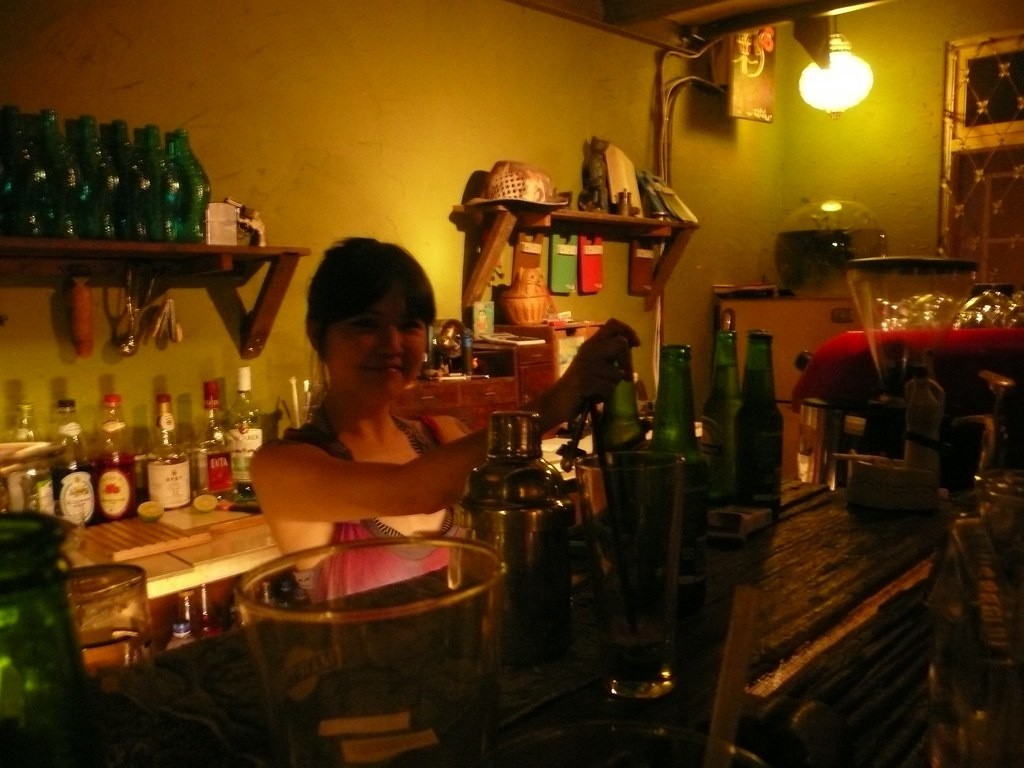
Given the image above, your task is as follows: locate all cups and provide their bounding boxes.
[470,721,770,768]
[234,536,509,768]
[575,453,687,706]
[62,564,156,679]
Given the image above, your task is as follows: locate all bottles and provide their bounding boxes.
[0,106,212,242]
[449,411,577,679]
[592,331,786,612]
[0,367,264,527]
[0,510,292,768]
[618,188,632,216]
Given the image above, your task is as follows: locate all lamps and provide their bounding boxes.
[799,15,873,119]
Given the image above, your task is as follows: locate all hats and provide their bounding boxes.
[466,160,569,208]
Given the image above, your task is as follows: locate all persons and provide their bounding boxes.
[250,237,640,602]
